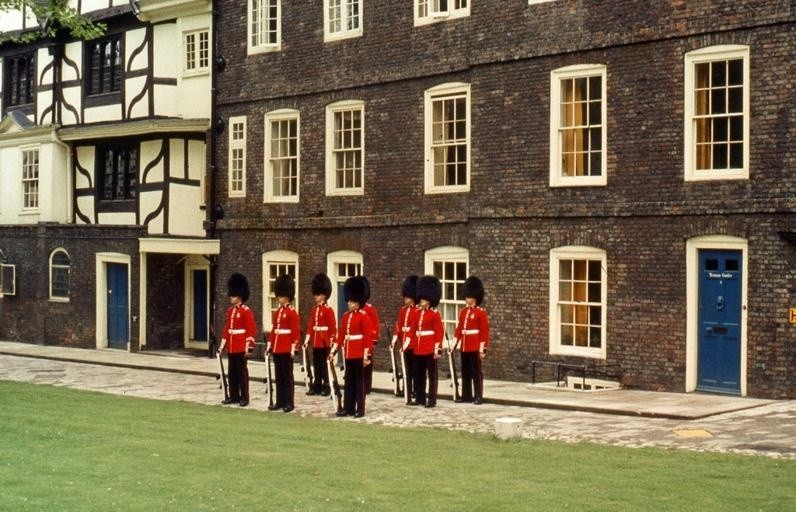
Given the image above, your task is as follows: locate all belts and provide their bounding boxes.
[313,326,328,331]
[402,326,411,332]
[416,330,435,336]
[228,329,246,335]
[461,329,480,335]
[274,329,291,334]
[345,334,363,340]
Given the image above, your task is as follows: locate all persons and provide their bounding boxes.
[216,295,256,407]
[398,299,443,409]
[300,293,336,397]
[326,301,373,419]
[446,296,490,403]
[387,296,415,396]
[359,303,379,399]
[263,296,302,413]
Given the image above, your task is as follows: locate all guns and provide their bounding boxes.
[327,335,342,416]
[385,322,400,396]
[211,325,229,403]
[300,329,312,394]
[445,331,459,402]
[262,330,273,407]
[398,329,412,404]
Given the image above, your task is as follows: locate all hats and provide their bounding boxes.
[403,275,441,306]
[344,276,370,308]
[228,272,249,302]
[312,273,331,299]
[274,275,294,301]
[463,276,484,305]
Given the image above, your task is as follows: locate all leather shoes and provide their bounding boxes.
[269,402,284,410]
[284,404,294,412]
[354,412,364,417]
[336,408,355,416]
[474,398,482,404]
[240,398,249,406]
[409,400,426,406]
[396,390,415,398]
[222,398,240,404]
[306,385,330,396]
[425,403,436,408]
[455,398,472,402]
